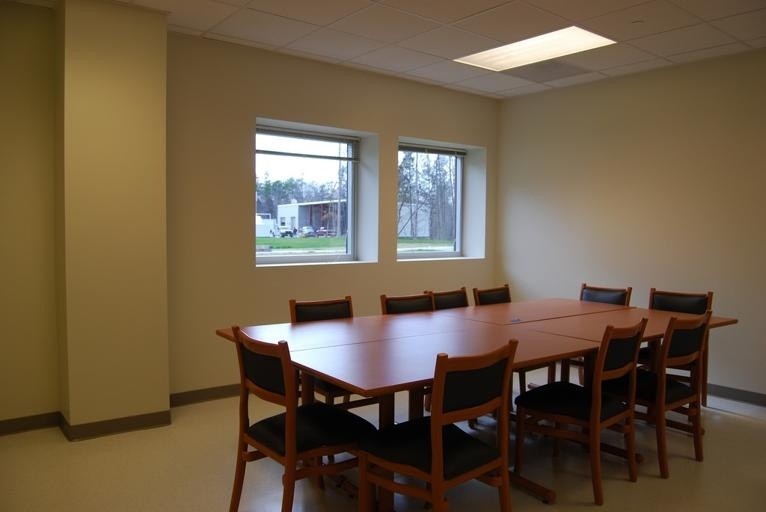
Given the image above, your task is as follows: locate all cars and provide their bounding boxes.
[269,223,334,240]
